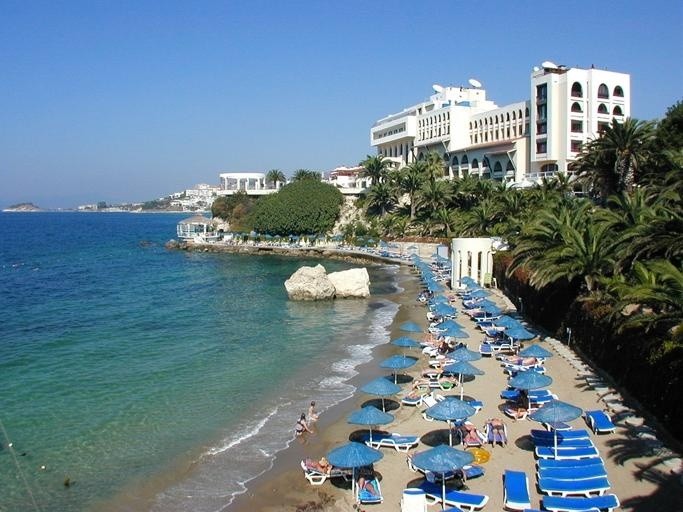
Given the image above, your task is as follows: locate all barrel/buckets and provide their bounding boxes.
[399,488,428,512]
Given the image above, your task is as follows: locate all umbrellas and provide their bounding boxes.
[213,229,388,246]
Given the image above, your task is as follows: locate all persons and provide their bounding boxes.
[310,458,333,475]
[307,399,319,421]
[293,412,313,436]
